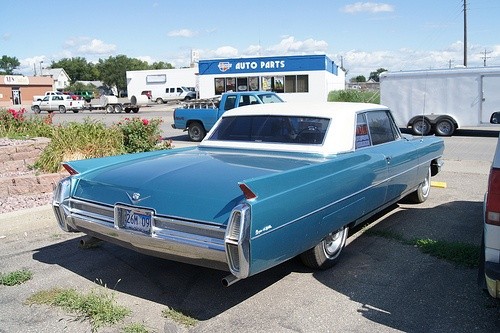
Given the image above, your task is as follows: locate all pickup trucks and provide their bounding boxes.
[172,91,285,142]
[33,91,64,101]
[31,95,85,114]
[152,86,193,104]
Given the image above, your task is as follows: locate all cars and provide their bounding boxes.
[50,101,445,289]
[479,111,500,299]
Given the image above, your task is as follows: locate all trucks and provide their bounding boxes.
[74,91,94,102]
[379,66,500,137]
